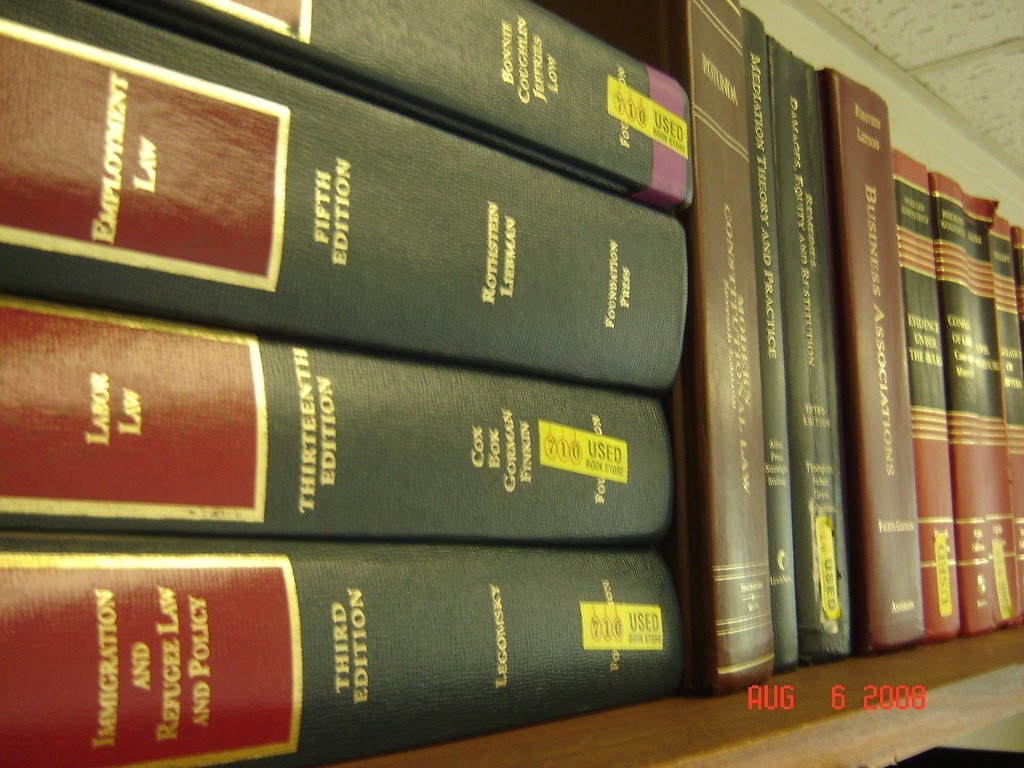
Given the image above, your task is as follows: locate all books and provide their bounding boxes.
[822,62,923,651]
[0,0,1024,768]
